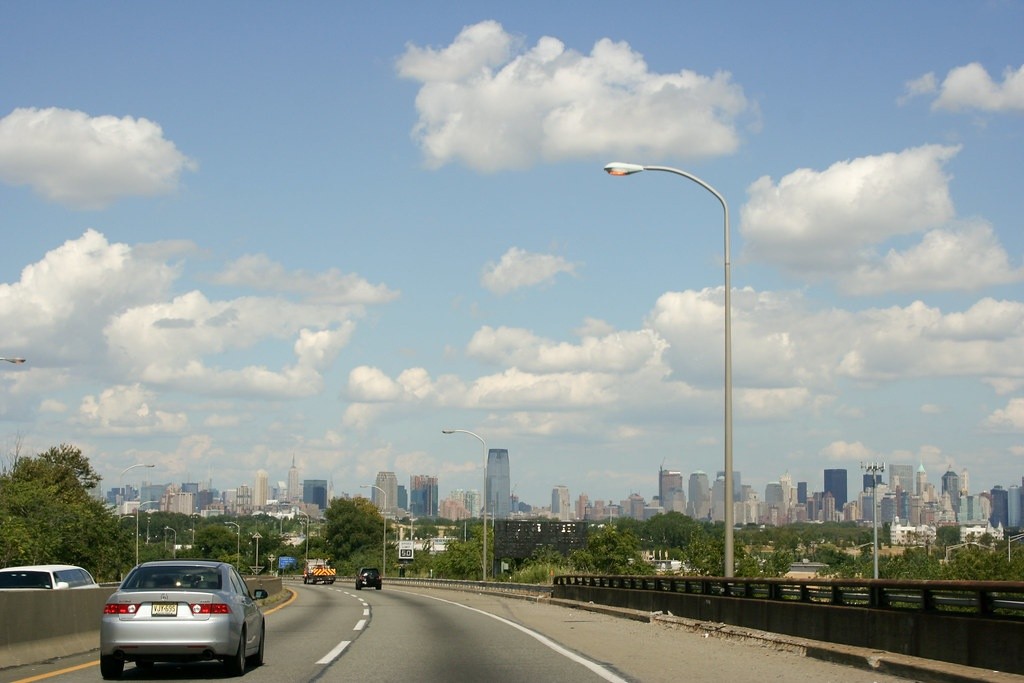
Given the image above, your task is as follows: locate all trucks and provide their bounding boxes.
[303,558,338,584]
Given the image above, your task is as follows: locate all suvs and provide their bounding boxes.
[355,567,382,590]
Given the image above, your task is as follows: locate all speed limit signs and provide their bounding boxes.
[399,541,414,559]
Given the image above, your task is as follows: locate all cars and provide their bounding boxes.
[100,561,268,678]
[0,565,99,590]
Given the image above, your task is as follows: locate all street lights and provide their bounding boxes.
[146,517,151,544]
[118,463,155,582]
[298,511,310,558]
[603,163,733,578]
[280,515,283,534]
[225,522,241,571]
[360,485,387,577]
[853,542,874,560]
[164,528,177,558]
[136,501,159,565]
[0,357,28,364]
[442,429,486,581]
[117,515,135,523]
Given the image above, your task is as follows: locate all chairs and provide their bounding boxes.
[143,576,176,587]
[195,581,217,588]
